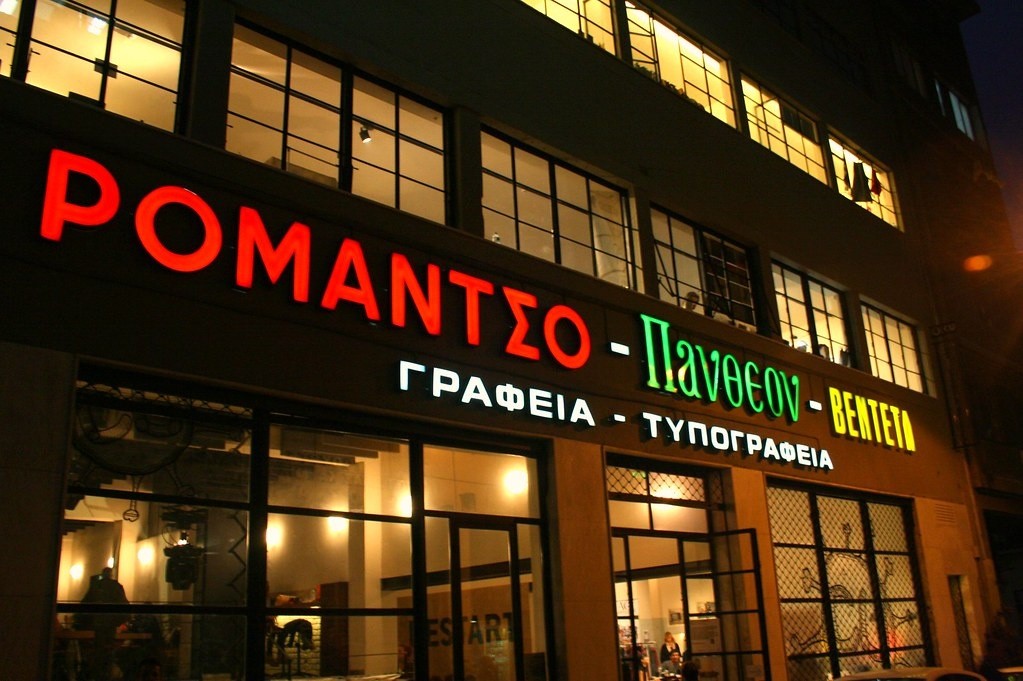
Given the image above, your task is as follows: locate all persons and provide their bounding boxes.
[681,291,700,310]
[985,609,1018,667]
[659,632,681,663]
[113,613,162,681]
[681,662,699,681]
[75,567,129,681]
[661,649,680,674]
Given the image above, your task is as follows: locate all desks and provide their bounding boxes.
[55,632,152,640]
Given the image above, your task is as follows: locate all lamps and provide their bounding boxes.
[806,371,822,412]
[359,125,376,143]
[600,308,631,356]
